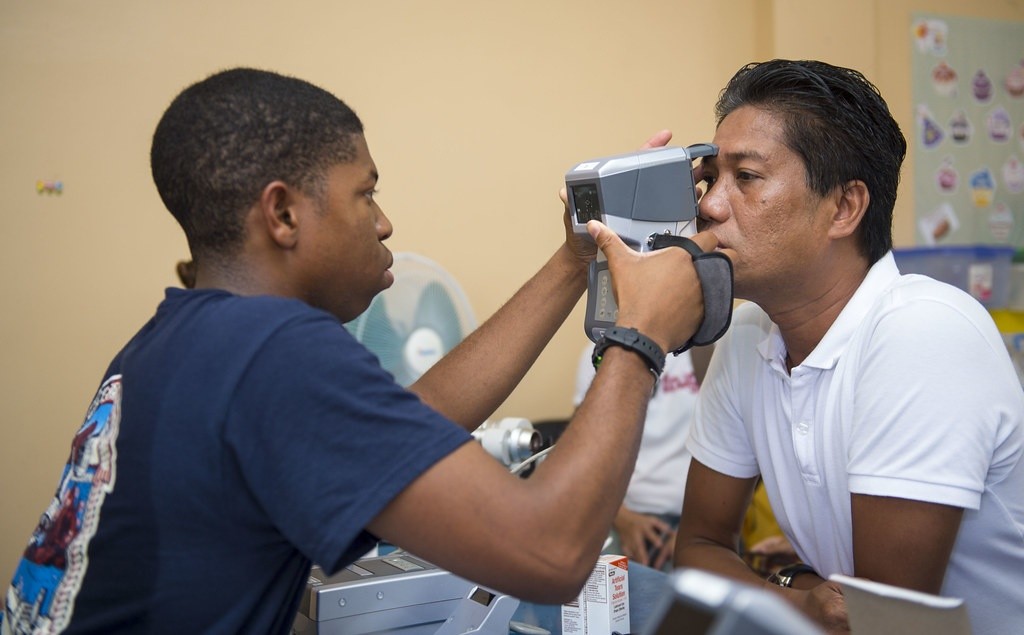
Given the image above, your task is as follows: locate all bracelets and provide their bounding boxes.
[592,326,665,384]
[771,564,817,588]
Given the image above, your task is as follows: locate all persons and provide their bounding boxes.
[576,345,745,565]
[0,69,705,635]
[674,58,1022,634]
